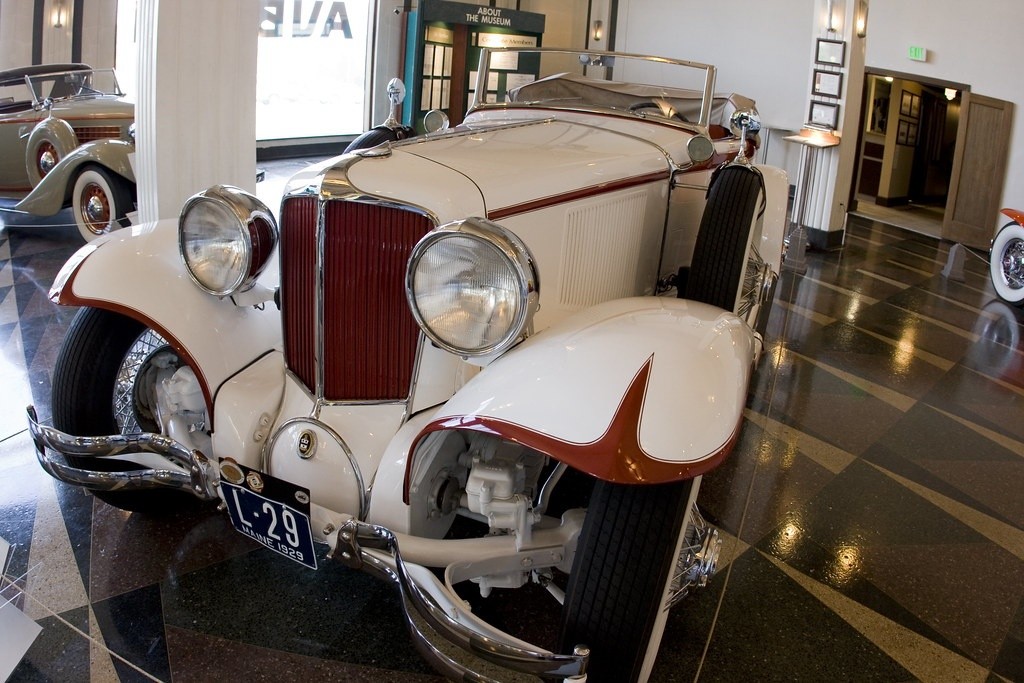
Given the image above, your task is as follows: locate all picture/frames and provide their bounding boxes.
[808,100,840,131]
[896,90,922,147]
[811,69,843,99]
[815,38,846,67]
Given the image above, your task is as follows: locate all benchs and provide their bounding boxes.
[508,71,758,140]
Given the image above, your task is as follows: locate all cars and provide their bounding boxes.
[26,43,793,683]
[0,63,265,245]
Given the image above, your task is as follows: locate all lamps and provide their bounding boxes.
[945,86,958,101]
[592,20,602,40]
[825,0,869,38]
[51,0,68,28]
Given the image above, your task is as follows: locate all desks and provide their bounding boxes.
[760,125,793,164]
[782,127,842,270]
